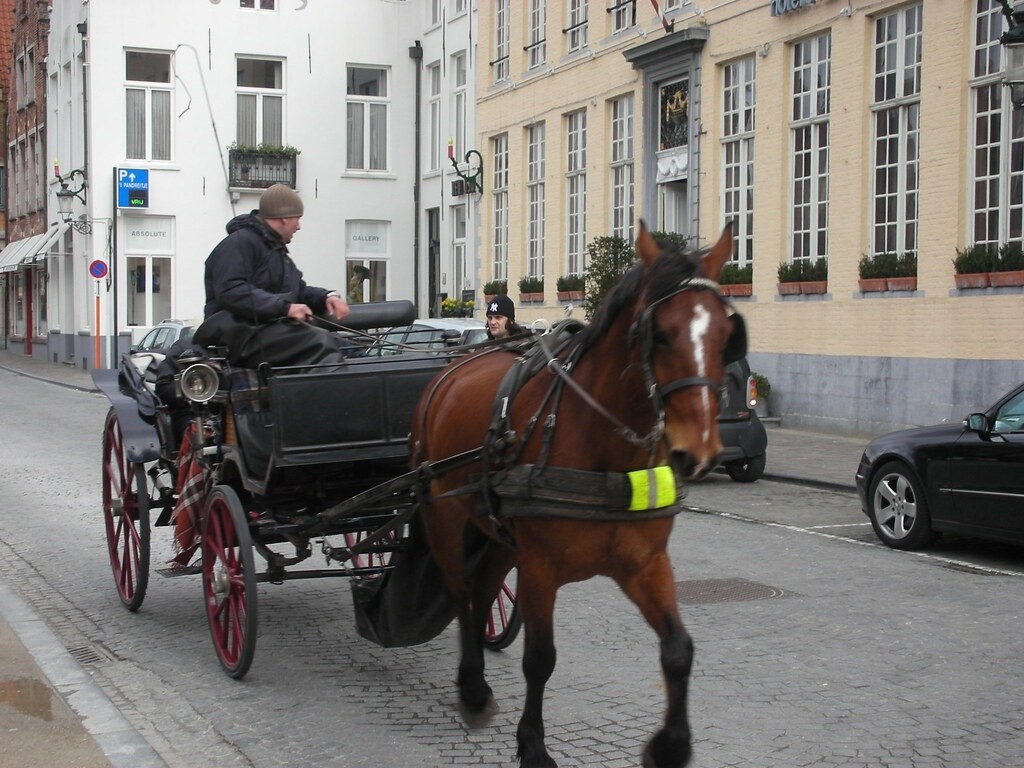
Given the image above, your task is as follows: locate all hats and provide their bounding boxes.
[259,184,303,219]
[486,295,514,319]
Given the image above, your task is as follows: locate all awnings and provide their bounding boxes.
[0,222,73,273]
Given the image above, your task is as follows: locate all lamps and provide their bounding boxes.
[54,183,92,235]
[447,139,483,197]
[53,156,87,205]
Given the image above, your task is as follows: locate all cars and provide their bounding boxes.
[853,381,1024,552]
[364,316,489,357]
[719,357,769,484]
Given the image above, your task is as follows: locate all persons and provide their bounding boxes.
[486,295,539,348]
[202,183,348,375]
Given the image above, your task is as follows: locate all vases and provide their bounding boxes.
[440,310,473,317]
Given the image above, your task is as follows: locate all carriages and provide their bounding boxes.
[88,223,749,768]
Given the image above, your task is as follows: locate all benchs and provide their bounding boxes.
[138,300,430,423]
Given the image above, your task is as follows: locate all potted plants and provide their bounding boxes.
[483,279,508,302]
[859,251,917,291]
[557,272,586,301]
[517,275,545,303]
[750,370,772,418]
[953,242,1023,289]
[716,261,754,296]
[230,142,298,181]
[776,257,829,296]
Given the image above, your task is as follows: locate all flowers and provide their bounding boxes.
[440,294,475,312]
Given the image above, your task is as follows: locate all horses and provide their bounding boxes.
[405,217,736,767]
